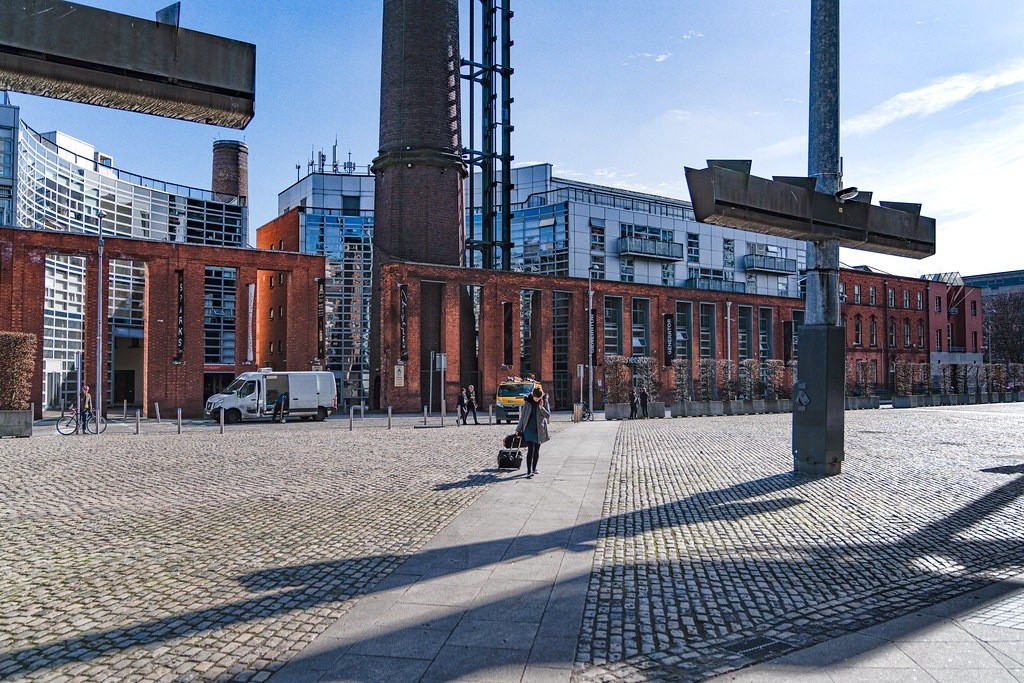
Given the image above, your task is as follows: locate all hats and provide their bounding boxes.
[533,388,543,398]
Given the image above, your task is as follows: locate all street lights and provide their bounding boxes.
[588,264,599,412]
[96,209,106,415]
[989,309,996,392]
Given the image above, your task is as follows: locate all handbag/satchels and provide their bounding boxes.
[503,433,527,448]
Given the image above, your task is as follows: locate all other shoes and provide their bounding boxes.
[534,470,539,474]
[529,472,534,476]
[83,430,89,434]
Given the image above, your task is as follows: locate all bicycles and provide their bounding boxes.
[571,401,595,422]
[56,408,108,435]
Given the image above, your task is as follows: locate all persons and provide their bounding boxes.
[456,385,480,426]
[516,387,551,478]
[628,387,640,420]
[271,392,288,423]
[69,385,92,434]
[1007,383,1012,392]
[640,388,649,419]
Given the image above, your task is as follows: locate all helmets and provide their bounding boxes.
[83,386,89,391]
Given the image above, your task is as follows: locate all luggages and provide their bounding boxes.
[497,436,522,470]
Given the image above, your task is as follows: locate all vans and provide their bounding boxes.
[496,378,544,425]
[205,367,338,422]
